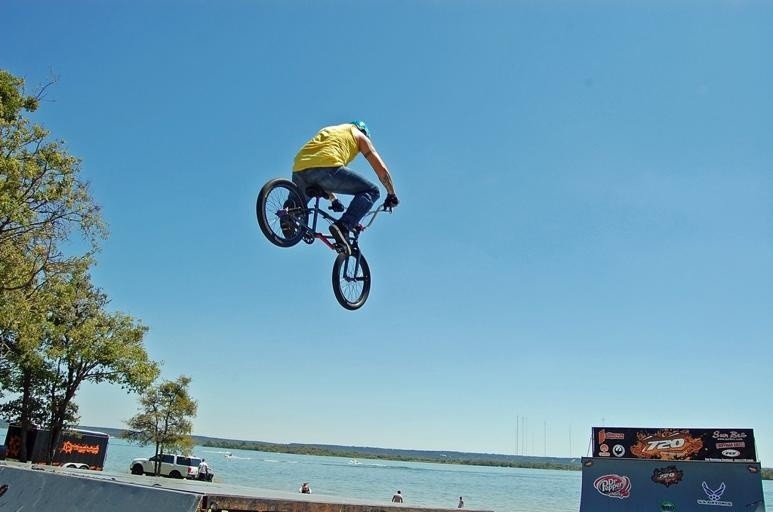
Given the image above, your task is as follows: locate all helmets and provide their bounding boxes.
[351,120,370,138]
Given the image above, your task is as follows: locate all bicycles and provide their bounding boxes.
[256,178,400,310]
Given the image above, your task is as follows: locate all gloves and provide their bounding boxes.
[385,194,398,207]
[332,199,344,212]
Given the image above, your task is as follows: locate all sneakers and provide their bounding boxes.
[329,220,352,256]
[280,199,297,239]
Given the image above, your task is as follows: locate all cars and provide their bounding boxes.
[130,453,202,479]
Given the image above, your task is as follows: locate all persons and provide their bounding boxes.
[299,481,312,494]
[457,496,464,508]
[392,490,404,503]
[280,119,399,257]
[198,458,207,479]
